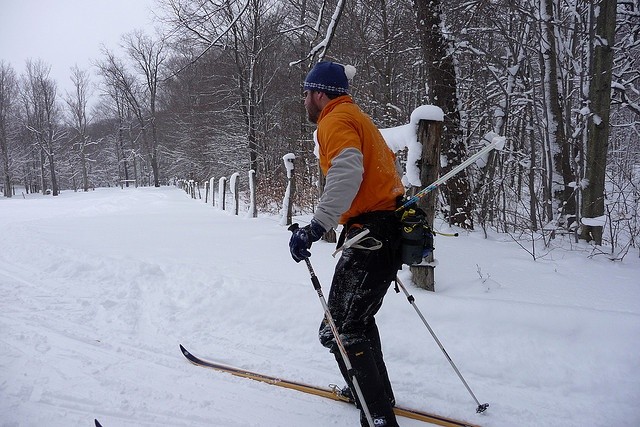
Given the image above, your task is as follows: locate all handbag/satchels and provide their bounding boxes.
[383,194,435,266]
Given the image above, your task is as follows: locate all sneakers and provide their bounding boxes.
[342,387,395,408]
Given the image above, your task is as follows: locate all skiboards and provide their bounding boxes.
[94,344,488,427]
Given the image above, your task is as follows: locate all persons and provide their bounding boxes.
[288,61,406,426]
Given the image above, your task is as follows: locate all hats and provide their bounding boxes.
[304,61,351,95]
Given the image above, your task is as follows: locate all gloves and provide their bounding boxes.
[289,219,327,263]
